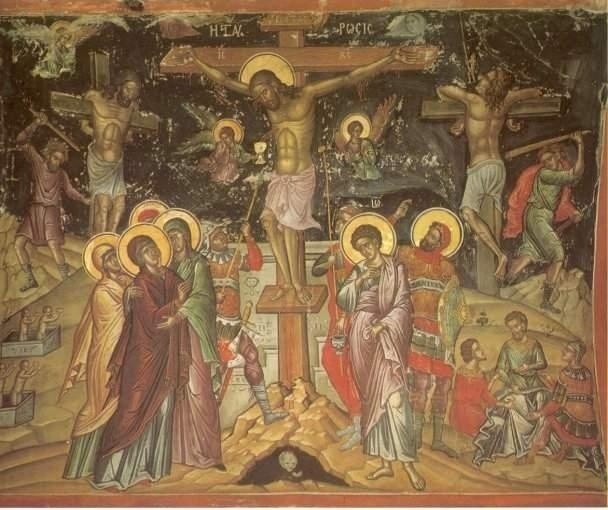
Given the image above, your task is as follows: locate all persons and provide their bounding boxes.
[180,37,426,305]
[78,69,150,239]
[197,128,258,184]
[447,337,514,451]
[71,245,134,484]
[392,219,465,461]
[114,236,192,486]
[337,223,431,494]
[489,311,553,422]
[514,335,601,466]
[198,215,292,427]
[335,116,380,181]
[438,63,543,281]
[154,218,229,474]
[503,127,592,312]
[15,107,95,295]
[138,210,163,223]
[306,198,415,456]
